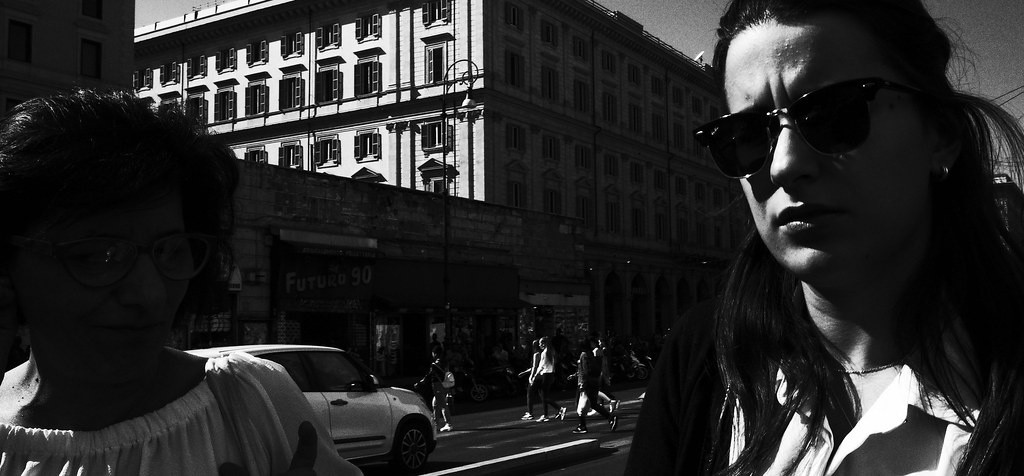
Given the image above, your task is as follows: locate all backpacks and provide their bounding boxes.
[432,361,456,389]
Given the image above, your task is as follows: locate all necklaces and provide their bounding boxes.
[837,331,921,374]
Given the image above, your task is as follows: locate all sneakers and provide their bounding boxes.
[587,409,601,416]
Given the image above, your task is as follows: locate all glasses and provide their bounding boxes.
[692,78,927,178]
[11,232,213,289]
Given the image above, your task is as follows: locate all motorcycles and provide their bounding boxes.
[605,328,651,385]
[451,360,520,402]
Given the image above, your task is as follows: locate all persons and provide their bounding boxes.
[0,88,361,476]
[413,330,625,434]
[624,0,1024,476]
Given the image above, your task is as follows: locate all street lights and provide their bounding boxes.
[438,57,483,373]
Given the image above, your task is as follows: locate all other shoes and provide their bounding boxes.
[610,415,617,431]
[555,407,566,420]
[440,423,454,431]
[535,414,547,422]
[572,425,587,434]
[521,411,534,420]
[609,399,621,414]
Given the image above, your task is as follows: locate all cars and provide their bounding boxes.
[179,344,438,475]
